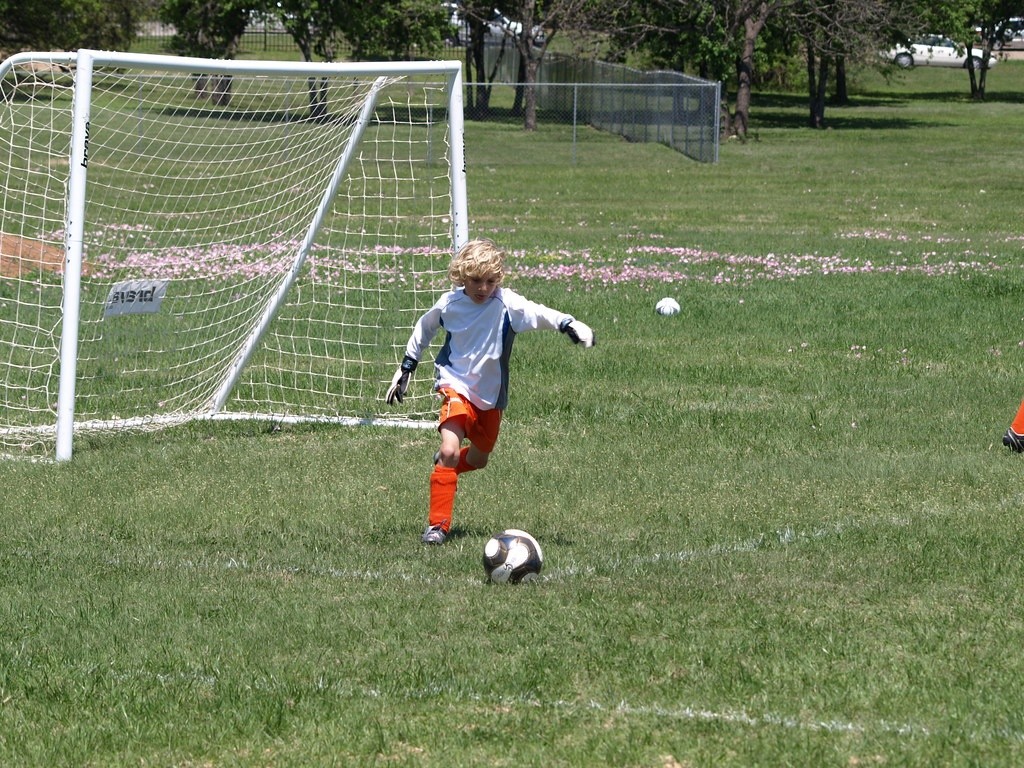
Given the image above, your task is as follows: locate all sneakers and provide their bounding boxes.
[434,450,441,465]
[424,525,446,545]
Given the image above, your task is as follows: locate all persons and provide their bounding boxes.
[386,236,596,545]
[1002,399,1024,454]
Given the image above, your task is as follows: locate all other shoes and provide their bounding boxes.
[1002,428,1024,454]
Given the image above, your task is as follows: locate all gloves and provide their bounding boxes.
[559,320,594,348]
[385,356,418,406]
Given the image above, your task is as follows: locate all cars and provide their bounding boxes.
[974,17,1012,51]
[877,32,999,71]
[439,3,547,48]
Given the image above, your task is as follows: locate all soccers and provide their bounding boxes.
[655,297,680,316]
[481,527,543,584]
